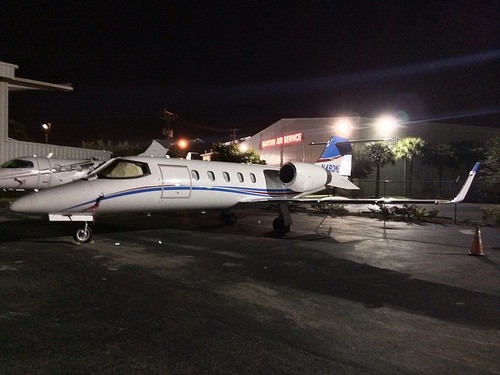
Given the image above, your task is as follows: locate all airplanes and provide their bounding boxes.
[0,139,199,195]
[9,136,482,244]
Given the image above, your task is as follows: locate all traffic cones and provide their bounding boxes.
[466,224,485,256]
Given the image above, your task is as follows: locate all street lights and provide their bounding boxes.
[41,122,52,144]
